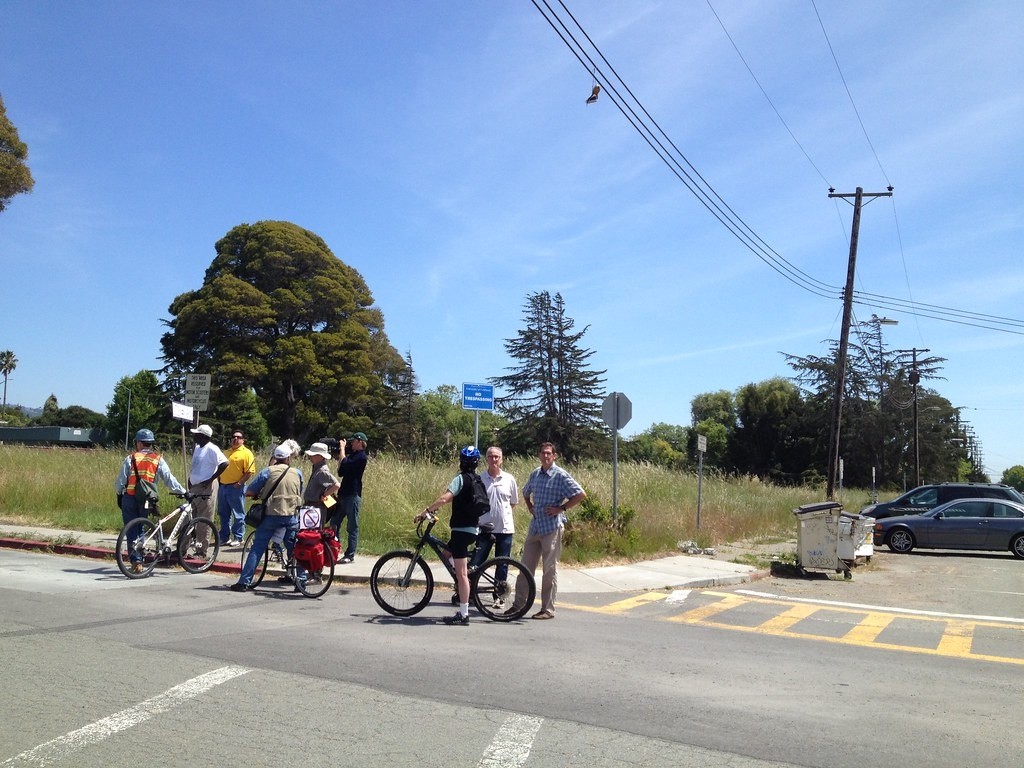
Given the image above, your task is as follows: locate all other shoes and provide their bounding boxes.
[169,549,188,559]
[187,552,207,559]
[337,556,351,563]
[230,539,244,546]
[130,565,142,573]
[492,598,505,608]
[230,583,246,592]
[294,581,306,592]
[278,576,292,583]
[504,607,518,614]
[219,539,231,545]
[532,611,554,619]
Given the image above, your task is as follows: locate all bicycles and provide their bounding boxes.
[240,495,336,598]
[370,511,537,623]
[116,493,220,580]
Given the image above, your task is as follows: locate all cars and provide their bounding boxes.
[872,495,1024,559]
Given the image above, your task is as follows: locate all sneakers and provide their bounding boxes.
[443,612,470,625]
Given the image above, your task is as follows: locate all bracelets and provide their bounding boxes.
[237,482,242,485]
[426,508,430,512]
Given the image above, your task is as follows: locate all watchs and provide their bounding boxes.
[561,505,566,511]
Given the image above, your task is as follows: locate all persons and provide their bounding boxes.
[504,443,588,620]
[116,424,367,593]
[452,446,518,608]
[415,444,481,625]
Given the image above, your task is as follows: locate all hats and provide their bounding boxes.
[275,444,293,458]
[190,424,214,438]
[347,432,368,441]
[305,442,331,460]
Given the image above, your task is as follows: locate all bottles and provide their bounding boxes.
[448,556,455,569]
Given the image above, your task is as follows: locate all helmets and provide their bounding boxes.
[136,429,155,442]
[460,445,480,463]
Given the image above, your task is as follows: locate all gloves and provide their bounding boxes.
[117,494,123,509]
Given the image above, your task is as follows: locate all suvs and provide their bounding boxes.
[859,481,1024,517]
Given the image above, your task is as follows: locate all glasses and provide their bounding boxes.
[231,436,243,439]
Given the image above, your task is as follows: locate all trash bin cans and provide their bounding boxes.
[791,501,852,581]
[839,511,876,568]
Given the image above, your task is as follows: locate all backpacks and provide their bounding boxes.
[464,472,490,517]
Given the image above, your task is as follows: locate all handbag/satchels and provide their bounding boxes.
[244,503,267,528]
[294,530,324,571]
[321,527,340,566]
[135,478,158,502]
